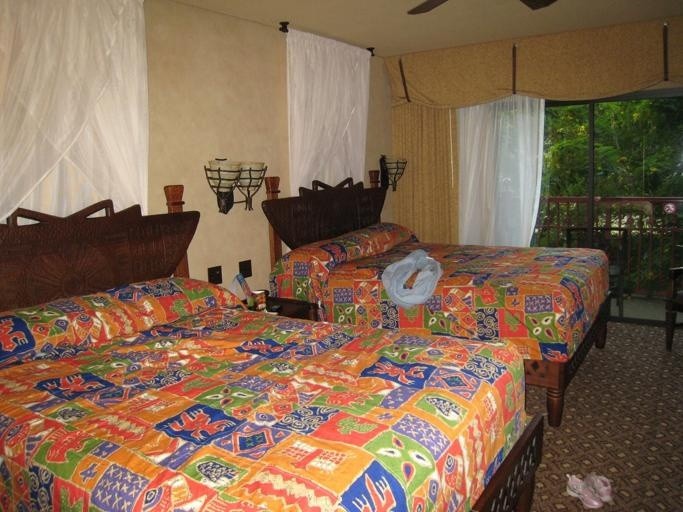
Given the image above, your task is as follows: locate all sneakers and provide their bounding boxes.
[566,471,613,509]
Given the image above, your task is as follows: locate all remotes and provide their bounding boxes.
[270,301,281,311]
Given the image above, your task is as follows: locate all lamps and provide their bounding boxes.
[378,154,408,192]
[203,157,270,216]
